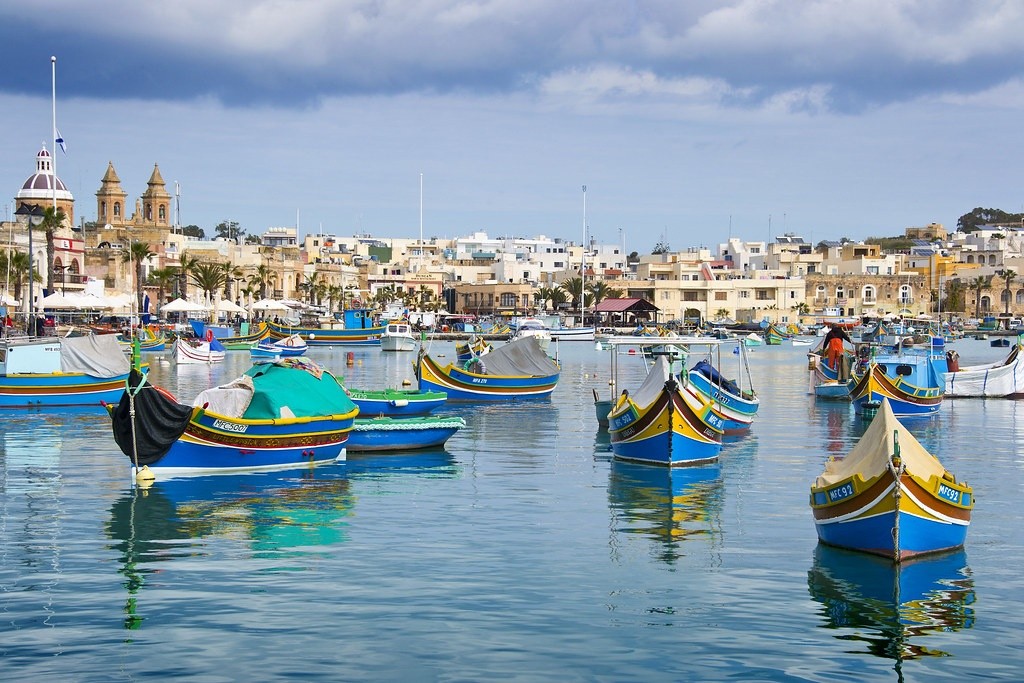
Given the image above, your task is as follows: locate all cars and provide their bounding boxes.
[667,319,682,327]
[962,322,978,331]
[949,321,964,332]
[867,320,878,328]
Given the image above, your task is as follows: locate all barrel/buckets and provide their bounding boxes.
[948,359,958,372]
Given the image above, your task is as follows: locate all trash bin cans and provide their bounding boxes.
[984,317,997,328]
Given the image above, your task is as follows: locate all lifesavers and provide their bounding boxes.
[206,329,213,342]
[286,338,294,348]
[351,299,362,309]
[851,361,869,376]
[463,357,485,375]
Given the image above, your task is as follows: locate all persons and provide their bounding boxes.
[415,318,422,332]
[36,317,54,337]
[2,315,13,327]
[823,323,852,384]
[274,315,278,323]
[267,315,271,321]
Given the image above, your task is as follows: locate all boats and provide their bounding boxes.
[380,323,417,352]
[99,342,362,479]
[809,397,977,564]
[267,306,391,347]
[169,328,227,365]
[990,336,1010,348]
[0,317,1024,469]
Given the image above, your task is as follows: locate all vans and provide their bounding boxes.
[1008,320,1022,330]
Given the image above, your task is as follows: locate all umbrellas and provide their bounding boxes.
[33,291,131,322]
[159,298,293,324]
